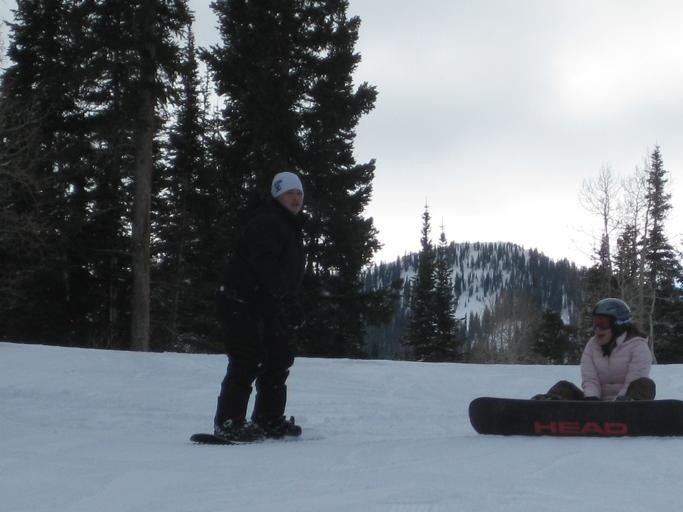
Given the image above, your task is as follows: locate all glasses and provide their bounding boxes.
[593,313,632,329]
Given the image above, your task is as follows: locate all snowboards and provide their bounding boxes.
[469,397,683,436]
[190,418,327,445]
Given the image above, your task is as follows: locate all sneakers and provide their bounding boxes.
[214,415,265,443]
[253,411,301,440]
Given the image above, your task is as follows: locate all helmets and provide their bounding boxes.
[592,298,632,331]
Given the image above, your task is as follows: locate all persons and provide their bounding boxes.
[214,171,304,442]
[533,299,655,403]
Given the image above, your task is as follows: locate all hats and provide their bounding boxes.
[271,172,304,199]
